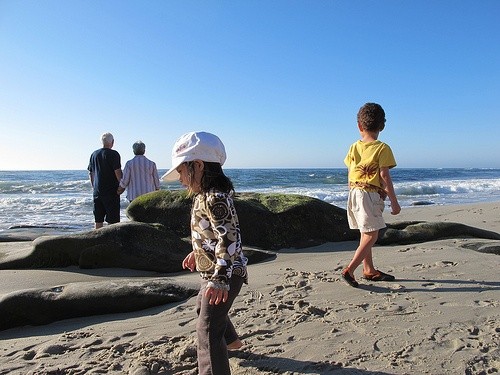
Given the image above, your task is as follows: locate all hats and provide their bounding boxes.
[161,131,226,182]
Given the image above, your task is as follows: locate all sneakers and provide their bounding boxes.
[363,269,395,281]
[341,267,359,288]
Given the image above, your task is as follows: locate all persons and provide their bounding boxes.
[87,133,124,229]
[117,140,160,203]
[340,103,401,288]
[159,131,248,375]
